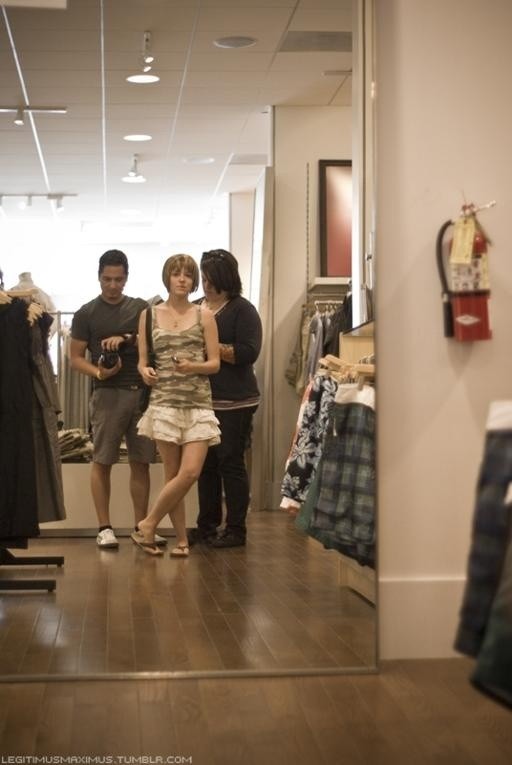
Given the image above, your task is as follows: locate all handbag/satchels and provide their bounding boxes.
[139,305,161,412]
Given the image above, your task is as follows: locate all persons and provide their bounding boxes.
[130,255,219,557]
[190,248,263,548]
[11,272,57,313]
[69,250,168,548]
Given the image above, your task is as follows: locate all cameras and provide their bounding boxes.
[101,349,118,369]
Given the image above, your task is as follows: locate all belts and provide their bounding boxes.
[118,384,141,391]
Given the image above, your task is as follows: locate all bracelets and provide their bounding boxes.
[97,370,105,381]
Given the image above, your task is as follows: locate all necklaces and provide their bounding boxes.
[168,308,186,328]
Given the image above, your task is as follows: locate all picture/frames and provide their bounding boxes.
[318,157,351,275]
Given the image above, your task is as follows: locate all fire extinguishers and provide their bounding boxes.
[435,199,498,343]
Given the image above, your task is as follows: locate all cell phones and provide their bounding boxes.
[172,357,179,363]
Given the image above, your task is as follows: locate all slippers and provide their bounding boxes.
[131,532,164,556]
[170,546,192,557]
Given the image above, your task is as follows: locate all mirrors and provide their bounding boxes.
[0,0,381,681]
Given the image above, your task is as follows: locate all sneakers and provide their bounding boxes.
[96,529,119,548]
[133,528,168,546]
[188,530,246,548]
